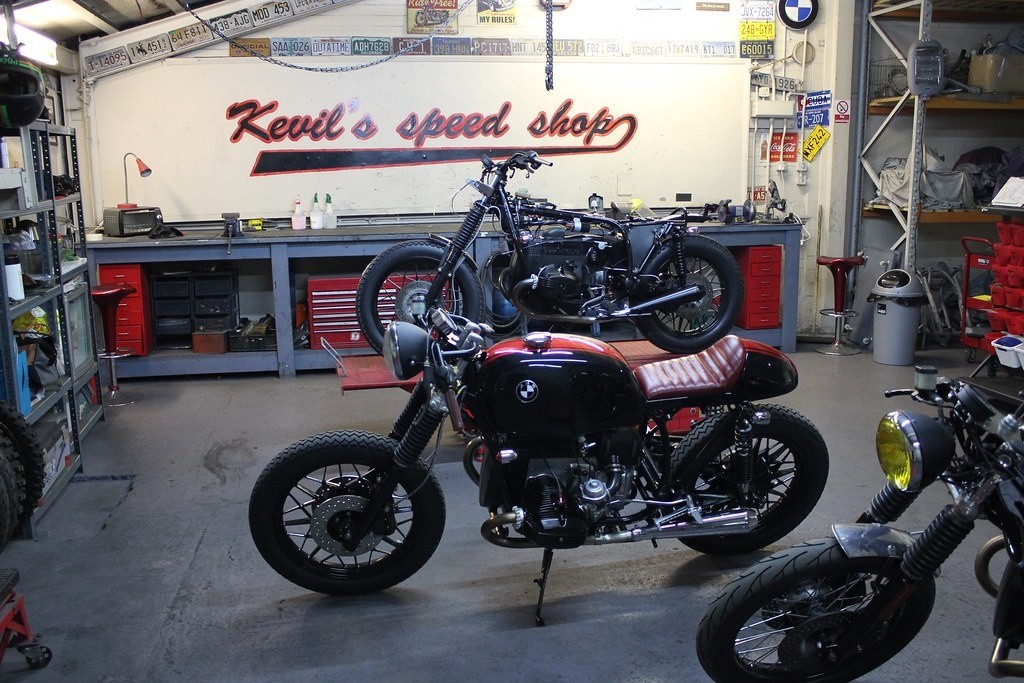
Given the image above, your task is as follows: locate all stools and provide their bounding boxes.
[90,282,139,408]
[813,253,867,356]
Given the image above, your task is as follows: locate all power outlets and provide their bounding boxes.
[778,159,789,172]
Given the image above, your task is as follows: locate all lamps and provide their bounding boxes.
[114,152,152,208]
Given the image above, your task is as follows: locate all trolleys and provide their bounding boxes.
[959,235,1000,377]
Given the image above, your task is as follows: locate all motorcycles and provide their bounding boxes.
[695,343,1024,683]
[356,145,746,369]
[247,308,831,628]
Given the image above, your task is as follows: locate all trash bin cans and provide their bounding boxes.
[872,269,926,366]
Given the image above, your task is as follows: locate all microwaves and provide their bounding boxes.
[103,206,163,236]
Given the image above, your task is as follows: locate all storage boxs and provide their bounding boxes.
[148,262,307,357]
[307,270,458,349]
[967,52,1024,95]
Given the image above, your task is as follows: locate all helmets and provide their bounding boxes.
[0,56,45,129]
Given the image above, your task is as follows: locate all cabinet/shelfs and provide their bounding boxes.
[839,1,1024,355]
[715,245,782,331]
[97,263,151,357]
[0,114,114,539]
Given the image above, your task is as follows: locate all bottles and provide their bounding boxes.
[4,254,25,300]
[588,193,603,212]
